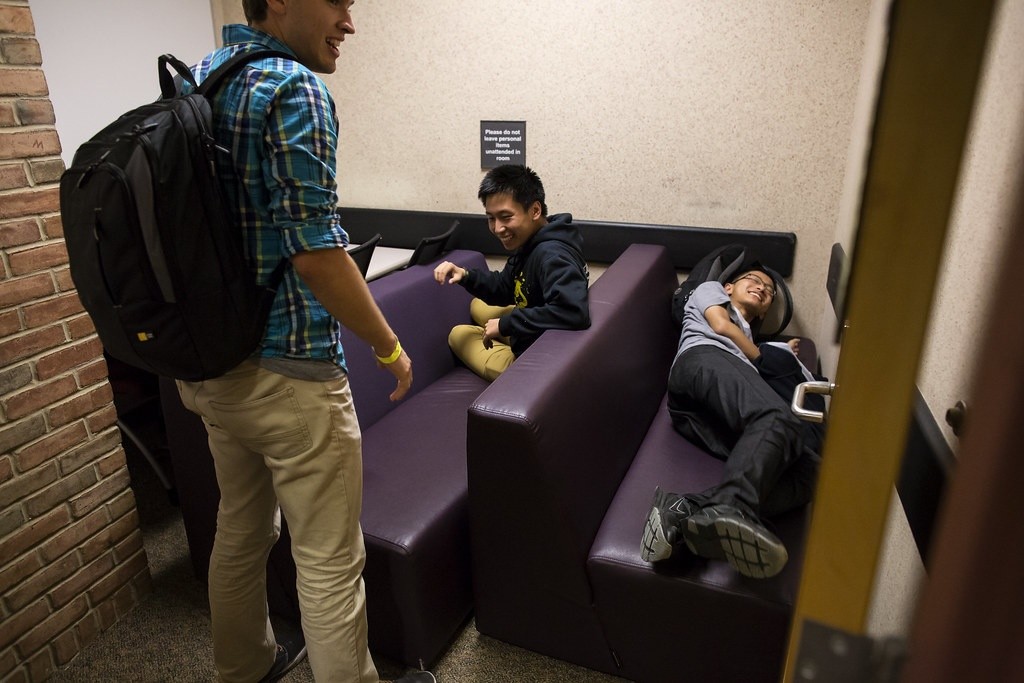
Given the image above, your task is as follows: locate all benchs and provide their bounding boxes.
[163,251,493,669]
[464,241,818,683]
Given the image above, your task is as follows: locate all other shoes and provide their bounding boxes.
[394,670,436,683]
[258,623,307,683]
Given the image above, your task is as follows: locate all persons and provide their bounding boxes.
[169,0,436,683]
[641,269,800,581]
[434,164,592,381]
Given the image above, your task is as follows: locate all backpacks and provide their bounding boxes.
[670,243,746,344]
[58,48,300,383]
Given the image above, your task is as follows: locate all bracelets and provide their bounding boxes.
[371,334,402,364]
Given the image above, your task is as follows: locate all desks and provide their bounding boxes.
[343,244,415,283]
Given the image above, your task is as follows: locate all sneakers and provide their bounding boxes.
[638,485,698,564]
[681,505,789,580]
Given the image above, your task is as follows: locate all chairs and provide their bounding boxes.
[405,221,461,269]
[346,233,382,279]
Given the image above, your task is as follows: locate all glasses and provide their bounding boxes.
[731,274,777,296]
